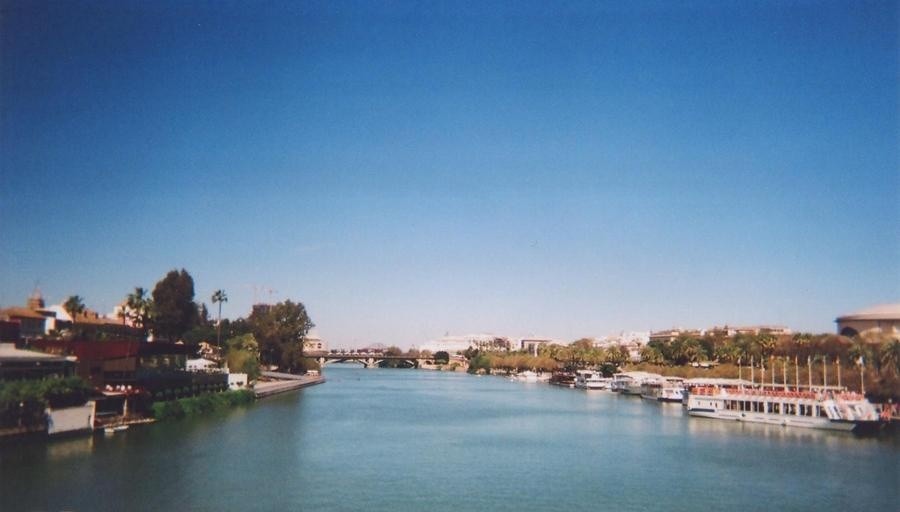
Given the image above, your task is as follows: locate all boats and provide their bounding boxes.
[683,382,881,432]
[104,425,129,434]
[549,368,686,402]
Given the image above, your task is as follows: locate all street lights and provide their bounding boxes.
[211,288,228,360]
